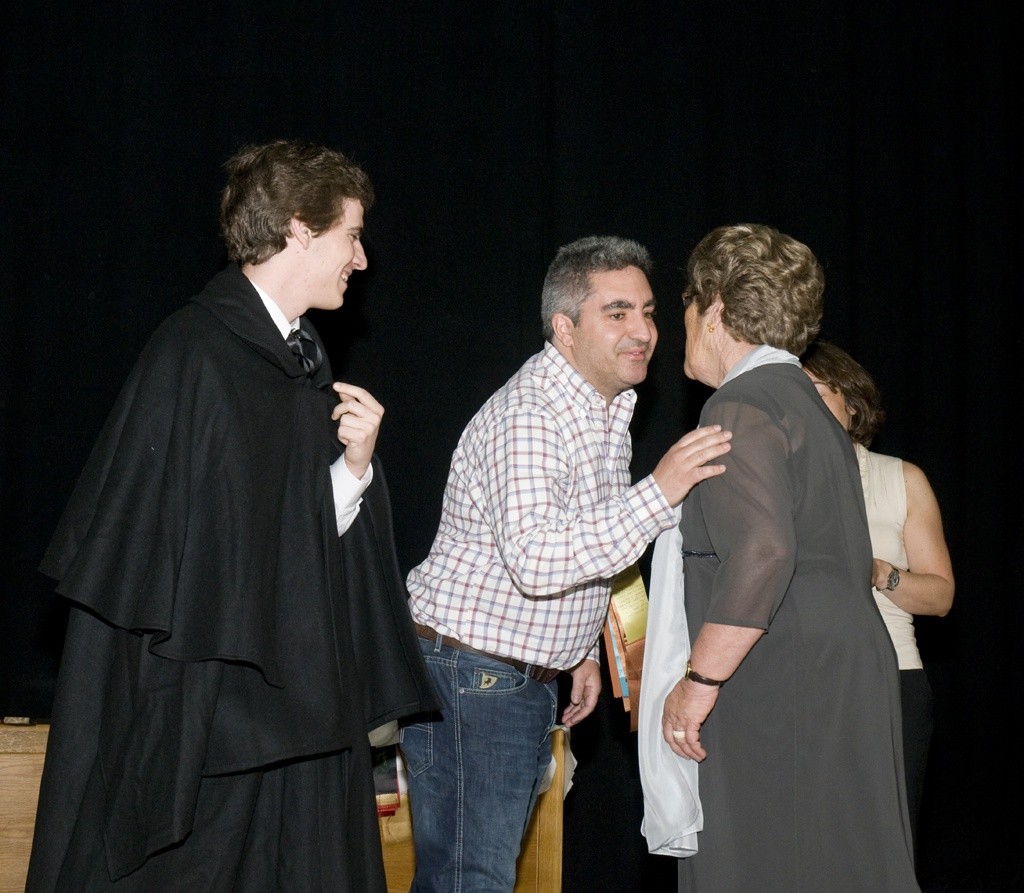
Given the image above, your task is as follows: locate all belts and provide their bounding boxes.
[414,622,561,682]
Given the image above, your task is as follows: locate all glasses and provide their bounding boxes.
[681,292,697,308]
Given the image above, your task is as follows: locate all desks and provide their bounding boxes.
[0,715,565,893]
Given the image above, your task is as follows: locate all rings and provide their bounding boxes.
[673,731,685,738]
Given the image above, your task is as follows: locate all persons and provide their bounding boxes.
[400,236,732,893]
[638,223,922,893]
[25,143,441,893]
[799,343,954,893]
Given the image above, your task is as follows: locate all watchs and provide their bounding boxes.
[685,661,721,686]
[876,564,900,594]
